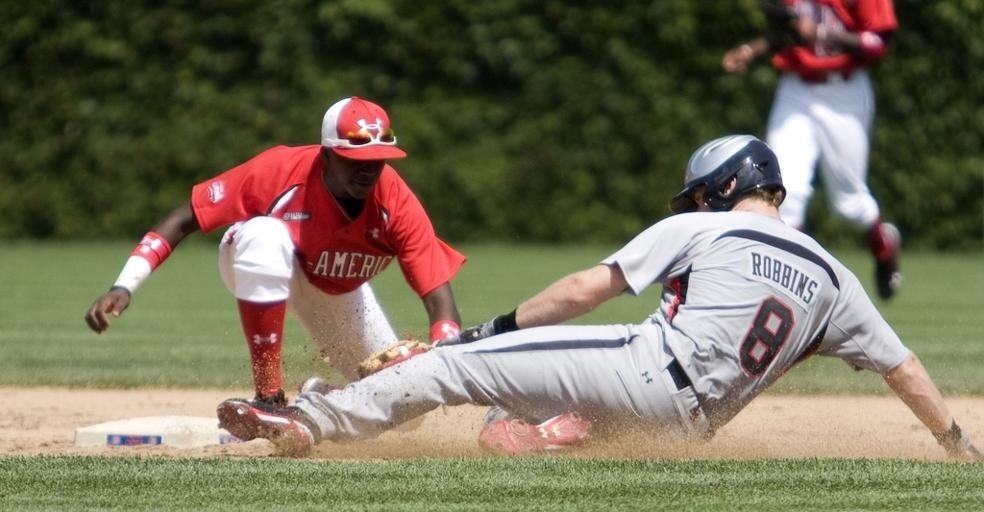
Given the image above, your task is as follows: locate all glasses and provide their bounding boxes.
[321,127,397,149]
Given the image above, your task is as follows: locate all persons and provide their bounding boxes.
[217,133,981,481]
[86,97,467,414]
[720,2,905,302]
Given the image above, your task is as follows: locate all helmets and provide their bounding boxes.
[670,134,786,214]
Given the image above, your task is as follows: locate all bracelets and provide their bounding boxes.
[110,230,172,296]
[428,319,461,346]
[815,21,828,42]
[740,43,754,63]
[935,418,964,448]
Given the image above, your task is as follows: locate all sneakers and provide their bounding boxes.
[864,217,902,301]
[478,411,593,458]
[217,376,330,456]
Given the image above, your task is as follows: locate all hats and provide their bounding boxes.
[321,95,407,161]
